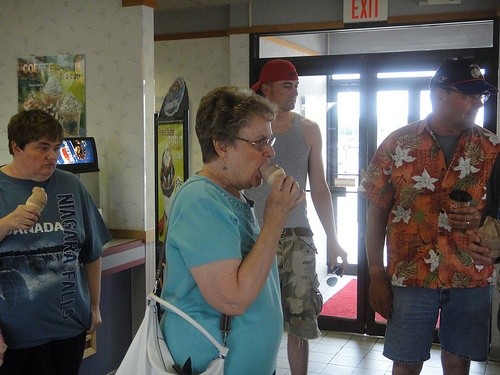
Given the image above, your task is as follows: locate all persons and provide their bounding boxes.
[360,60,500,375]
[467,151,500,265]
[241,59,347,375]
[160,85,305,375]
[0,110,113,375]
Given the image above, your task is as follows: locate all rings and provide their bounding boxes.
[467,221,470,225]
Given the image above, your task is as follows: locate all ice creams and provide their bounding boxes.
[160,149,175,218]
[41,76,81,137]
[26,186,47,213]
[259,162,286,192]
[477,216,500,258]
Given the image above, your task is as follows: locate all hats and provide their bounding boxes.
[251,59,298,92]
[429,60,498,95]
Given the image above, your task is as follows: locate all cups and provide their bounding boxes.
[449,189,472,213]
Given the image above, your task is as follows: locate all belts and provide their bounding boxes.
[281,227,314,237]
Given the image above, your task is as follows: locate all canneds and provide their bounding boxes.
[56,139,78,165]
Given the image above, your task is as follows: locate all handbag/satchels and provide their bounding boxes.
[114,180,236,375]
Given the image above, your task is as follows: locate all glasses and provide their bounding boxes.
[456,93,490,105]
[234,137,277,152]
